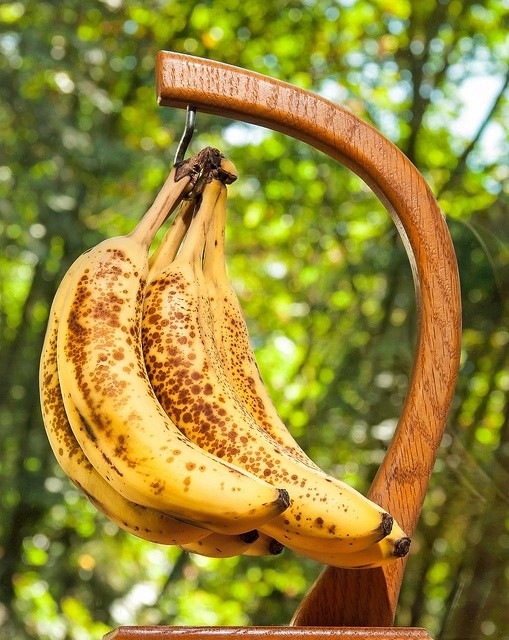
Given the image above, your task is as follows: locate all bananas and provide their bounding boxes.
[39,146,411,571]
